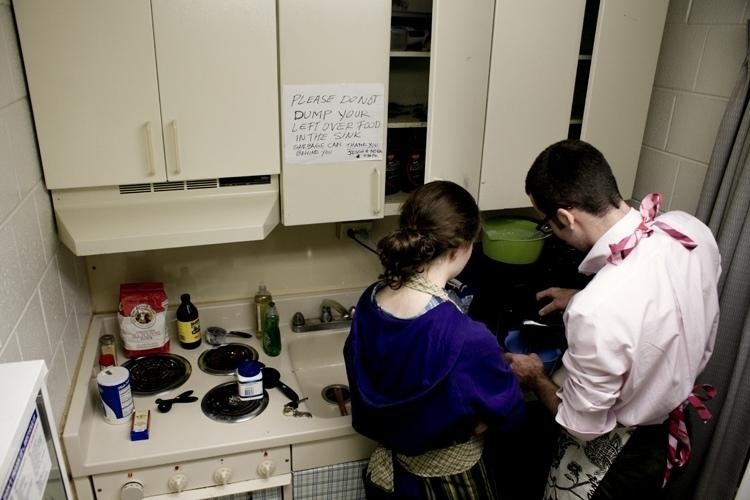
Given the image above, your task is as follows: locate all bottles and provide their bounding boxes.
[386,154,402,195]
[403,154,424,193]
[96,282,281,426]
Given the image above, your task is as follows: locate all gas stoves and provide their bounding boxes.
[85,330,292,500]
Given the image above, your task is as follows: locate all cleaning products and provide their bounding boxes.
[254,282,273,339]
[263,302,281,357]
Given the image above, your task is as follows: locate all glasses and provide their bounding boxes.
[537,205,573,233]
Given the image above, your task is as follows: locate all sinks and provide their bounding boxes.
[287,329,352,419]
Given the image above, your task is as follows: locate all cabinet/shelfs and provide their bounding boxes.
[11,1,281,257]
[278,1,497,226]
[476,1,671,211]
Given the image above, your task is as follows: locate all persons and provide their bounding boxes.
[343,180,522,498]
[505,139,723,499]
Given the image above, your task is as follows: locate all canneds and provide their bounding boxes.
[96,366,135,426]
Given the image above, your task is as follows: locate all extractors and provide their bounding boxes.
[51,175,281,257]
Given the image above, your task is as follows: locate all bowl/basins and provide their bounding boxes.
[482,219,548,264]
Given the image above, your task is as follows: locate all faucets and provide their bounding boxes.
[321,298,348,322]
